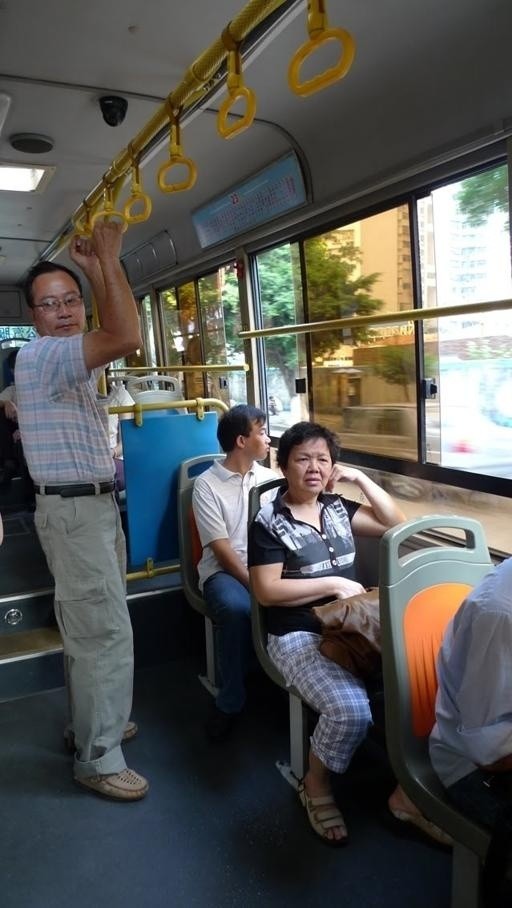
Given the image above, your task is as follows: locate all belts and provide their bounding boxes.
[32,481,120,498]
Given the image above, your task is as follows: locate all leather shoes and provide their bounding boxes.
[63,721,140,747]
[75,771,152,800]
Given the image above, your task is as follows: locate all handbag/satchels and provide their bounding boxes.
[311,585,384,689]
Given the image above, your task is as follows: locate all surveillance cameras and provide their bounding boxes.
[98,95,129,128]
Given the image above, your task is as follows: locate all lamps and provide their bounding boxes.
[11,133,55,154]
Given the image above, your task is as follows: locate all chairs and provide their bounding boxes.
[1,338,39,468]
[177,453,238,701]
[248,477,380,806]
[377,514,510,907]
[126,376,190,420]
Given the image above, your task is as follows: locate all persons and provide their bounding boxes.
[0,350,17,419]
[106,362,136,499]
[185,337,211,399]
[248,422,405,844]
[193,405,283,710]
[268,396,283,415]
[15,220,148,798]
[428,555,512,908]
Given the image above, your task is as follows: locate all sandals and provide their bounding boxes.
[386,808,454,847]
[294,783,347,842]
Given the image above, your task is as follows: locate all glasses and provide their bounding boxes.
[31,296,83,313]
